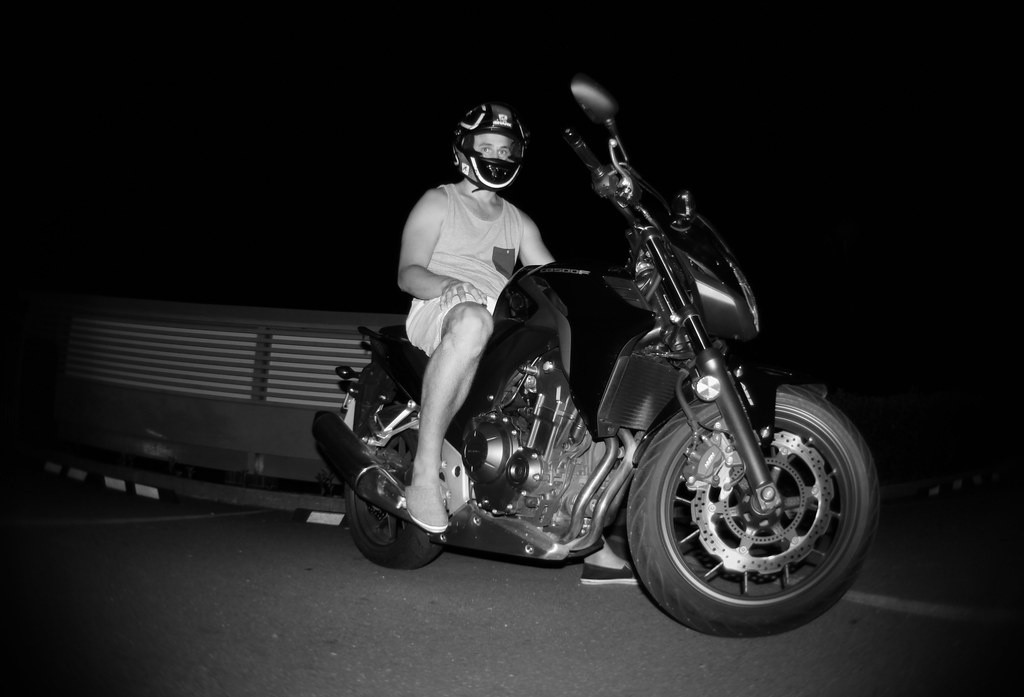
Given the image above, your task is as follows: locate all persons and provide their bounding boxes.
[397,101,570,534]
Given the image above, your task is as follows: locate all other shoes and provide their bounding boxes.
[406,485,449,534]
[580,562,638,585]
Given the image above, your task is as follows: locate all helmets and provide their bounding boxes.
[453,104,525,191]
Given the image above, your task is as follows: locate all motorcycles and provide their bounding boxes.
[311,81,885,640]
